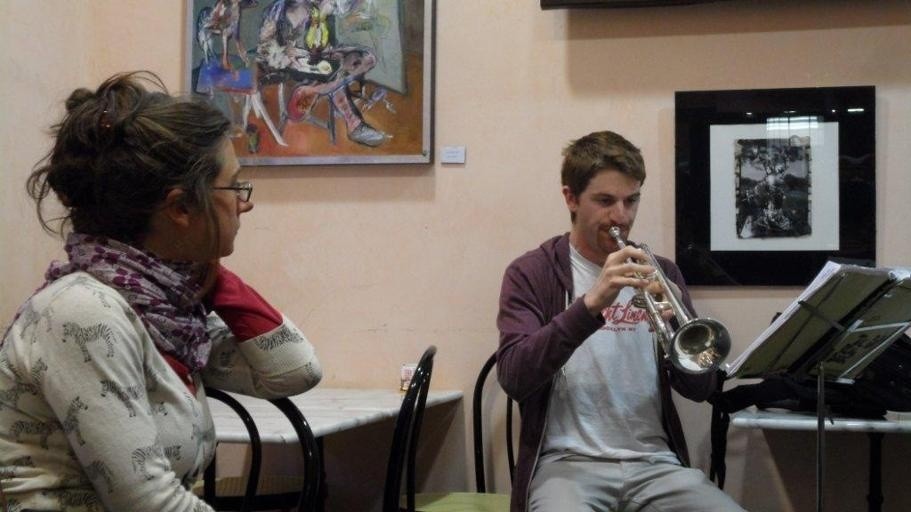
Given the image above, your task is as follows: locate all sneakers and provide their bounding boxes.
[347,121,385,146]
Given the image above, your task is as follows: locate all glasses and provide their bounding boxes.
[183,181,252,203]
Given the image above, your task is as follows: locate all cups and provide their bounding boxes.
[400,364,420,394]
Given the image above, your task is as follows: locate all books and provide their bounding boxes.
[725,259,911,383]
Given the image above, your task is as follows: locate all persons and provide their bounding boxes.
[489,127,762,511]
[252,0,388,151]
[0,68,328,512]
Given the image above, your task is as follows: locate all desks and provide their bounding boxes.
[732,403,911,510]
[201,387,464,510]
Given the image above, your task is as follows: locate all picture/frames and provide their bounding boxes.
[183,1,438,169]
[674,83,877,289]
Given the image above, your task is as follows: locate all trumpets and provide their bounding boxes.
[608,226,731,379]
